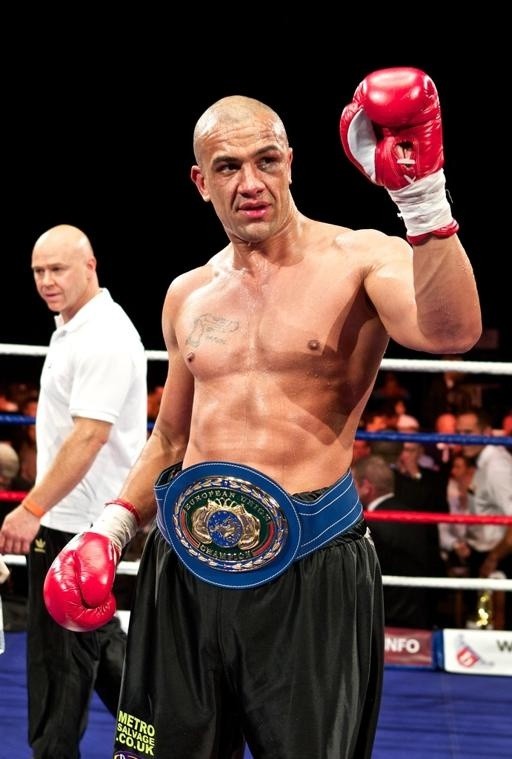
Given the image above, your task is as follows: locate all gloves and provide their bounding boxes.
[43,497,142,632]
[341,66,460,246]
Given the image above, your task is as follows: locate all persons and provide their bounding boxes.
[40,66,484,758]
[0,223,148,759]
[0,327,512,630]
